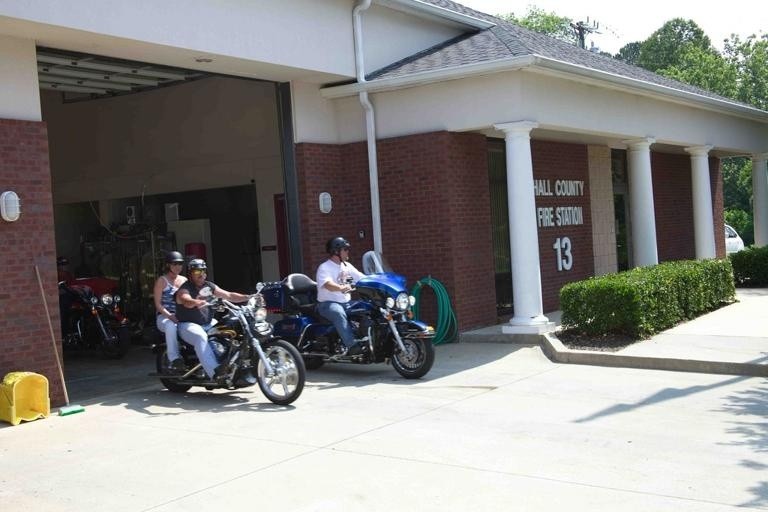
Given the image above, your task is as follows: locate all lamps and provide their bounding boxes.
[319,192,332,214]
[0,191,21,223]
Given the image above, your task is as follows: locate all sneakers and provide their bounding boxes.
[172,359,183,366]
[350,345,362,354]
[213,364,225,379]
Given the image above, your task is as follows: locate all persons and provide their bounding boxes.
[152,250,188,369]
[172,259,256,383]
[316,236,368,355]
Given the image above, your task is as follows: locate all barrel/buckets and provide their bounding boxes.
[0,370,51,427]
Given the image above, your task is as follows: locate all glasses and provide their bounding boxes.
[192,269,207,275]
[339,247,350,252]
[172,263,182,266]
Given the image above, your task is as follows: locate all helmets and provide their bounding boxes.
[326,237,350,255]
[188,259,207,271]
[167,251,184,263]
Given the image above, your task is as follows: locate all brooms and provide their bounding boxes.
[35,264,86,414]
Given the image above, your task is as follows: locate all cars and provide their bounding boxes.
[724,224,745,259]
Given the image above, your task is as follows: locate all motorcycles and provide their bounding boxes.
[259,251,436,380]
[149,282,305,405]
[59,280,130,355]
